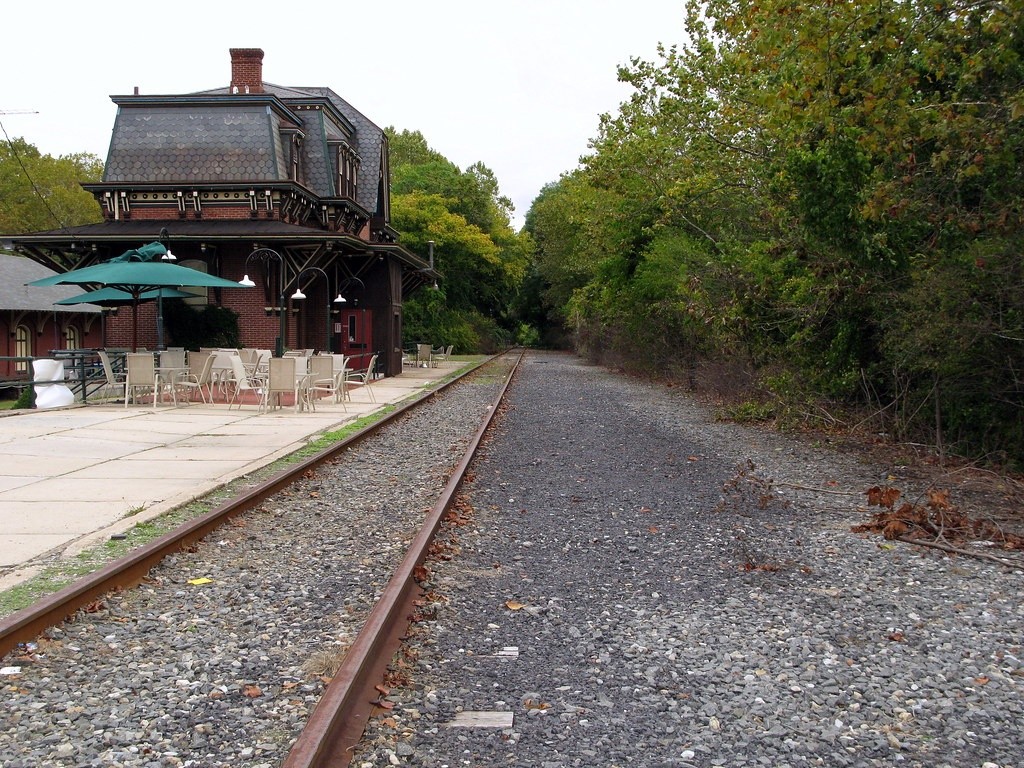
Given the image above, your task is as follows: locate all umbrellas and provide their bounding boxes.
[53,285,207,308]
[24,240,254,354]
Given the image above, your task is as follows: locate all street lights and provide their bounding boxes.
[333,275,366,382]
[238,247,286,406]
[290,267,332,394]
[155,227,178,367]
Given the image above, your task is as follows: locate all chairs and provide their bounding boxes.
[402,345,454,369]
[97,347,380,415]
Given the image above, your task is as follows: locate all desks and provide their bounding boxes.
[124,367,191,408]
[308,368,354,403]
[256,372,319,414]
[244,362,269,373]
